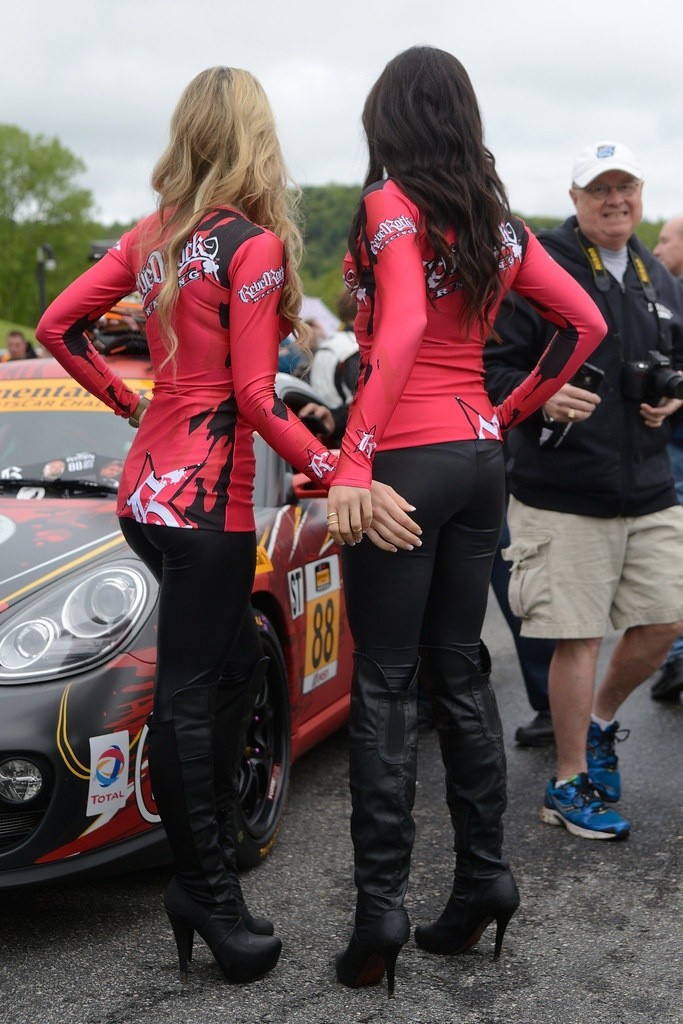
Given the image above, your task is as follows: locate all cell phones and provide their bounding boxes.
[569,362,604,395]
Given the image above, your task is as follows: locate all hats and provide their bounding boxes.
[569,142,643,189]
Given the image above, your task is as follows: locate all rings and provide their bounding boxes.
[327,512,336,519]
[353,528,363,533]
[326,521,339,525]
[568,409,575,421]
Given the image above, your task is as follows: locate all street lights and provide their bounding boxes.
[37,245,55,316]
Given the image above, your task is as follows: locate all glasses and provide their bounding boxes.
[573,181,639,200]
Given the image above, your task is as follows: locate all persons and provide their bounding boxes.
[35,66,422,984]
[299,142,683,838]
[326,46,608,993]
[6,329,32,361]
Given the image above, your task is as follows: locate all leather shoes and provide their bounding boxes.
[515,709,556,748]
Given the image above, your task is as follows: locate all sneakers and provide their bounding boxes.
[540,772,630,839]
[585,720,630,803]
[650,640,683,698]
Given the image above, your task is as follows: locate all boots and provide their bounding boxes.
[336,639,521,999]
[145,657,283,982]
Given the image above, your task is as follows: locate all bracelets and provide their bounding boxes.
[128,396,151,428]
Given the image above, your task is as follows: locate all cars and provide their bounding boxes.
[0,358,349,887]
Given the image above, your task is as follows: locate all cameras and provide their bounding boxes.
[623,350,683,408]
